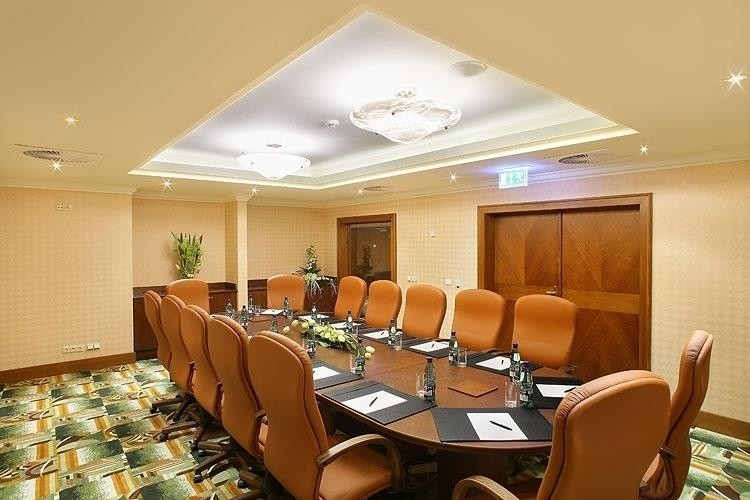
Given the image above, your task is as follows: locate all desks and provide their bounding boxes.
[212,304,586,500]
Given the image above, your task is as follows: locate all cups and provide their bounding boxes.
[255,305,260,316]
[395,336,402,351]
[352,326,359,336]
[458,347,467,367]
[287,307,293,319]
[504,382,518,408]
[415,372,425,399]
[350,356,355,373]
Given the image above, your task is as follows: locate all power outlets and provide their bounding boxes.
[61,344,85,354]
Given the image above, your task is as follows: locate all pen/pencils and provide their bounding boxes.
[343,322,347,325]
[381,332,385,335]
[271,309,275,312]
[312,370,315,374]
[564,386,576,392]
[431,343,434,348]
[368,395,378,407]
[501,360,504,365]
[490,419,513,431]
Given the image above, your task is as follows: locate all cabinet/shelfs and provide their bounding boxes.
[133,281,237,361]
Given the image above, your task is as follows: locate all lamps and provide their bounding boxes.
[350,85,461,144]
[236,143,312,180]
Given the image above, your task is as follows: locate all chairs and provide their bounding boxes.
[151,296,189,427]
[451,368,674,500]
[451,288,506,350]
[159,306,222,455]
[403,284,446,338]
[366,280,402,329]
[193,315,270,488]
[641,329,713,500]
[246,331,407,500]
[266,274,306,311]
[332,276,366,321]
[166,278,212,313]
[144,290,178,416]
[512,293,578,368]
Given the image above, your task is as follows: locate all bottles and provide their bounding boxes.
[519,360,534,409]
[271,315,278,332]
[423,357,437,403]
[355,338,366,375]
[448,331,459,367]
[240,305,248,329]
[226,299,233,318]
[311,303,317,320]
[510,344,521,382]
[283,297,289,316]
[307,325,316,356]
[388,319,397,350]
[248,295,255,317]
[346,310,353,333]
